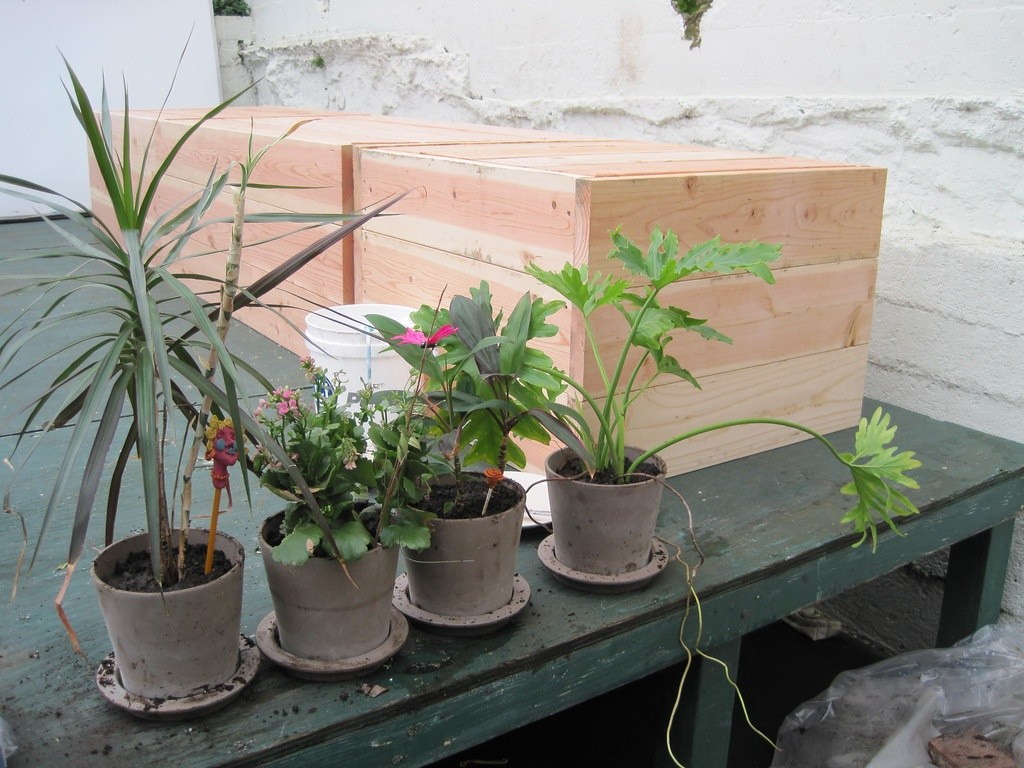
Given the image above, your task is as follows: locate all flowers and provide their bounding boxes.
[224,321,498,570]
[0,19,363,591]
[371,302,561,517]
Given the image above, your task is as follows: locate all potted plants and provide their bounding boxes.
[492,223,923,589]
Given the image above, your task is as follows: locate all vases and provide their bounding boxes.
[90,527,261,715]
[256,503,409,675]
[389,474,530,629]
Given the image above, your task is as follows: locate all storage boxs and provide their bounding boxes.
[161,118,614,365]
[354,143,890,481]
[89,108,385,278]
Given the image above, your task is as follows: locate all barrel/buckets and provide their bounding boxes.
[304,303,426,459]
